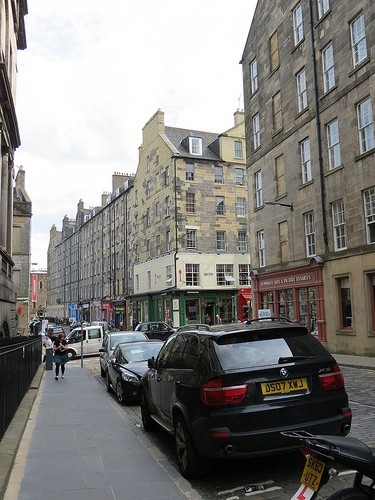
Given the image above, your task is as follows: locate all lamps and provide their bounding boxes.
[264,201,294,212]
[166,277,173,284]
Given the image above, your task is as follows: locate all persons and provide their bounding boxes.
[53,333,68,380]
[205,313,210,324]
[29,322,57,362]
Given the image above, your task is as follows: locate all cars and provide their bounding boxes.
[31,317,107,343]
[105,339,165,404]
[98,330,149,379]
[133,321,177,340]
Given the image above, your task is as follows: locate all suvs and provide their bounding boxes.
[139,316,353,478]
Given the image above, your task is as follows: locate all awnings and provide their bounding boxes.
[241,293,252,299]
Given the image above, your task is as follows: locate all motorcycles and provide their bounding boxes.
[279,430,375,500]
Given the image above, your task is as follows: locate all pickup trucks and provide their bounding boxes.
[64,325,105,360]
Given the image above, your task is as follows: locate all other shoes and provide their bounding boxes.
[61,375,64,378]
[55,376,59,380]
